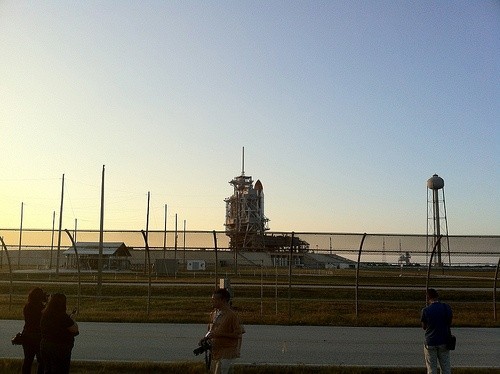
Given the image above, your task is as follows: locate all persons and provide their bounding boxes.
[38,293,79,374]
[21,287,47,374]
[421,289,453,374]
[199,289,246,374]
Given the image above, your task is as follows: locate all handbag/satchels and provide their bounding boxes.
[449,335,456,351]
[12,332,24,347]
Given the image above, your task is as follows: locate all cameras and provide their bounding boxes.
[12,332,23,345]
[193,341,211,356]
